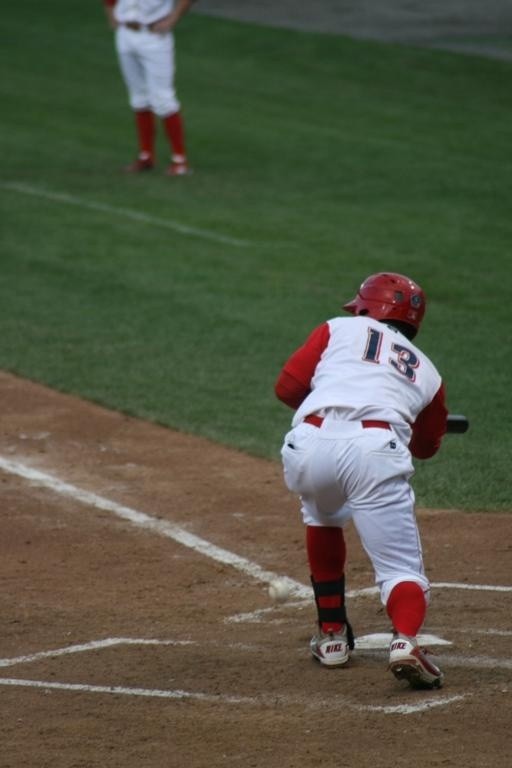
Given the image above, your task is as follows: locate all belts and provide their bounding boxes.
[305,415,391,431]
[125,22,152,31]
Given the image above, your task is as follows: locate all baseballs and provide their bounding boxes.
[267,581,290,603]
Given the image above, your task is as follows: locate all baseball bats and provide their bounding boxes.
[445,416,468,432]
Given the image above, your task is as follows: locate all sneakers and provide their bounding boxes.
[388,637,446,689]
[127,155,189,176]
[309,631,351,668]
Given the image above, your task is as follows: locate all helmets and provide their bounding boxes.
[342,272,426,330]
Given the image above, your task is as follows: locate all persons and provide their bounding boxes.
[105,0,193,176]
[275,273,448,689]
